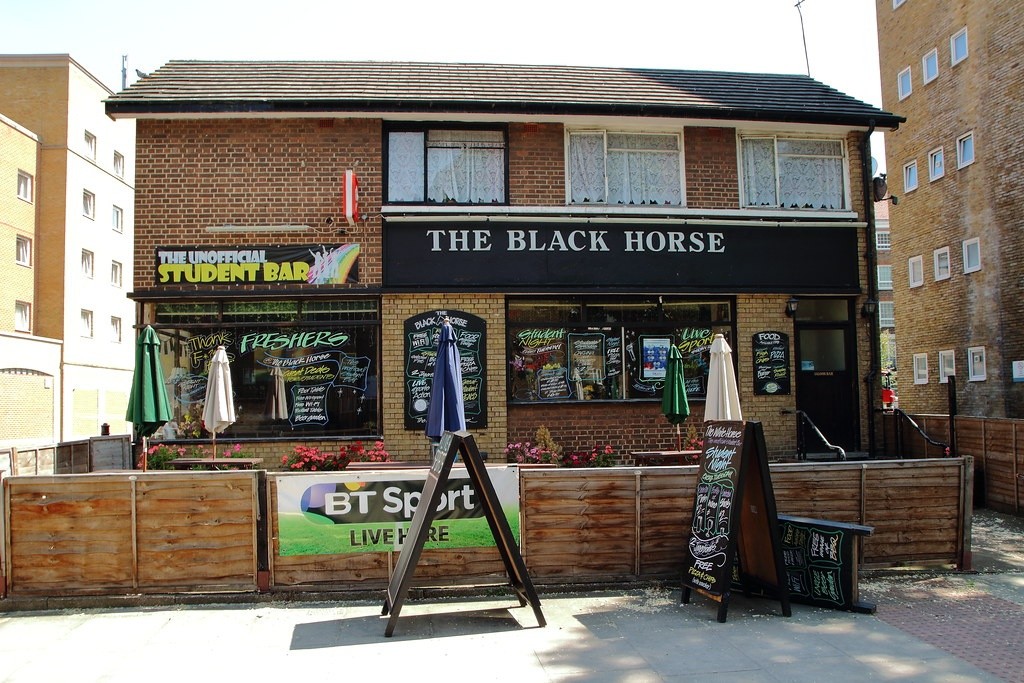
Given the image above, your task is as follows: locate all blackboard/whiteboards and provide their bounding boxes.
[729,514,856,613]
[680,419,753,604]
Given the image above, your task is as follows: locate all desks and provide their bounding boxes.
[630,450,703,467]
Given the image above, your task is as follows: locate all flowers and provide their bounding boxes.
[137,444,257,469]
[683,426,704,466]
[564,446,616,468]
[279,442,392,472]
[505,441,559,464]
